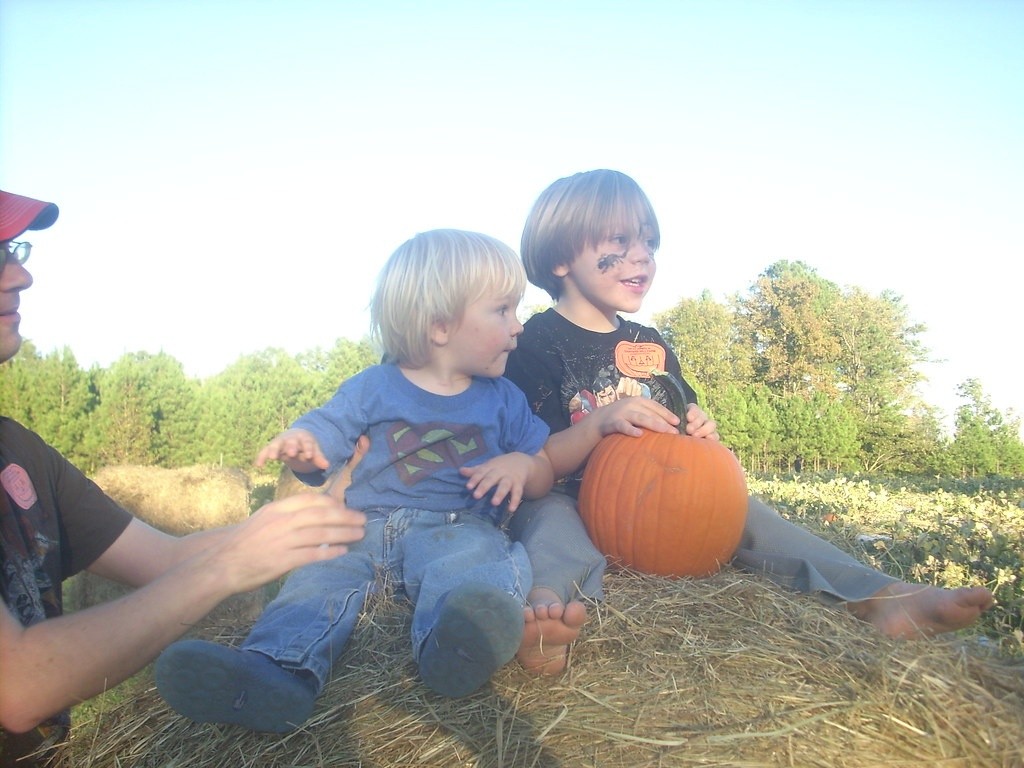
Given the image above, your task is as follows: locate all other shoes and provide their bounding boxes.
[154,640,312,733]
[419,583,523,698]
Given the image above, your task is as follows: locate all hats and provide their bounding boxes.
[0,190,59,241]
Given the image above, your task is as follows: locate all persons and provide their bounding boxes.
[155,228,555,734]
[502,169,996,675]
[0,189,368,768]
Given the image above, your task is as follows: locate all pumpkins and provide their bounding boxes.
[577,368,748,580]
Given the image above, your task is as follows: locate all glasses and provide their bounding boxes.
[0,241,32,273]
